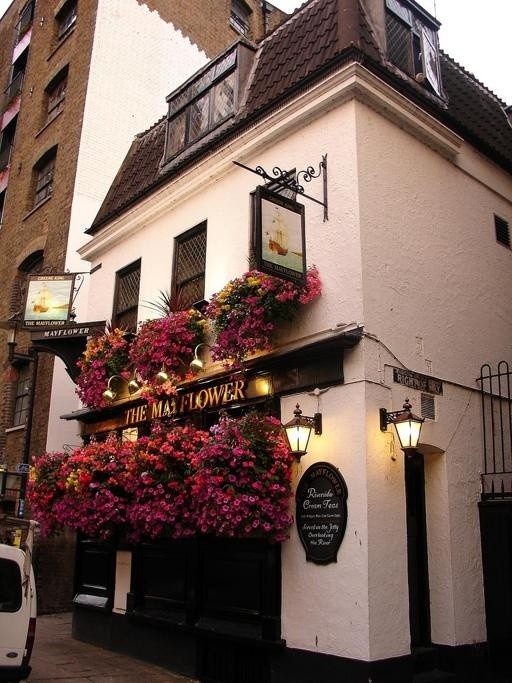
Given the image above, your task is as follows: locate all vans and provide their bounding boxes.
[1,545,37,679]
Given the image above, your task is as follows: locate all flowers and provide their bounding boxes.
[23,410,293,546]
[202,265,320,373]
[72,305,203,411]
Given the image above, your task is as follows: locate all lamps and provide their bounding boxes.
[378,393,427,460]
[282,403,324,465]
[189,341,218,373]
